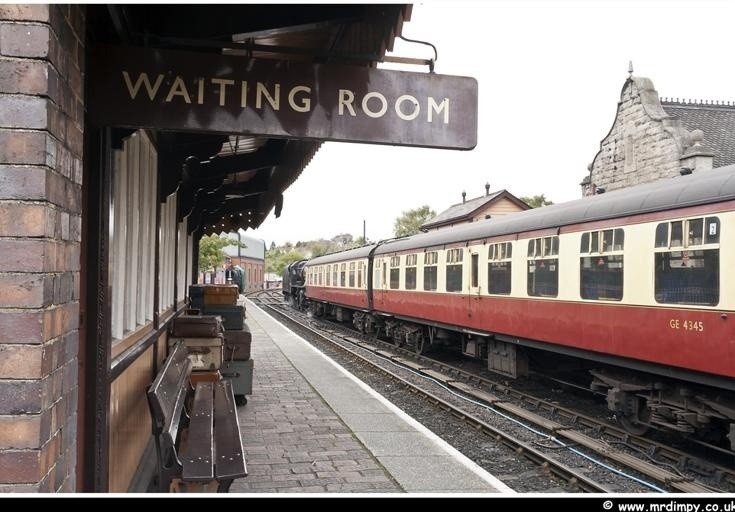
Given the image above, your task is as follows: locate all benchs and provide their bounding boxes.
[145,338,248,493]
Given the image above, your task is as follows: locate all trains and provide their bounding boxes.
[282,165,735,455]
